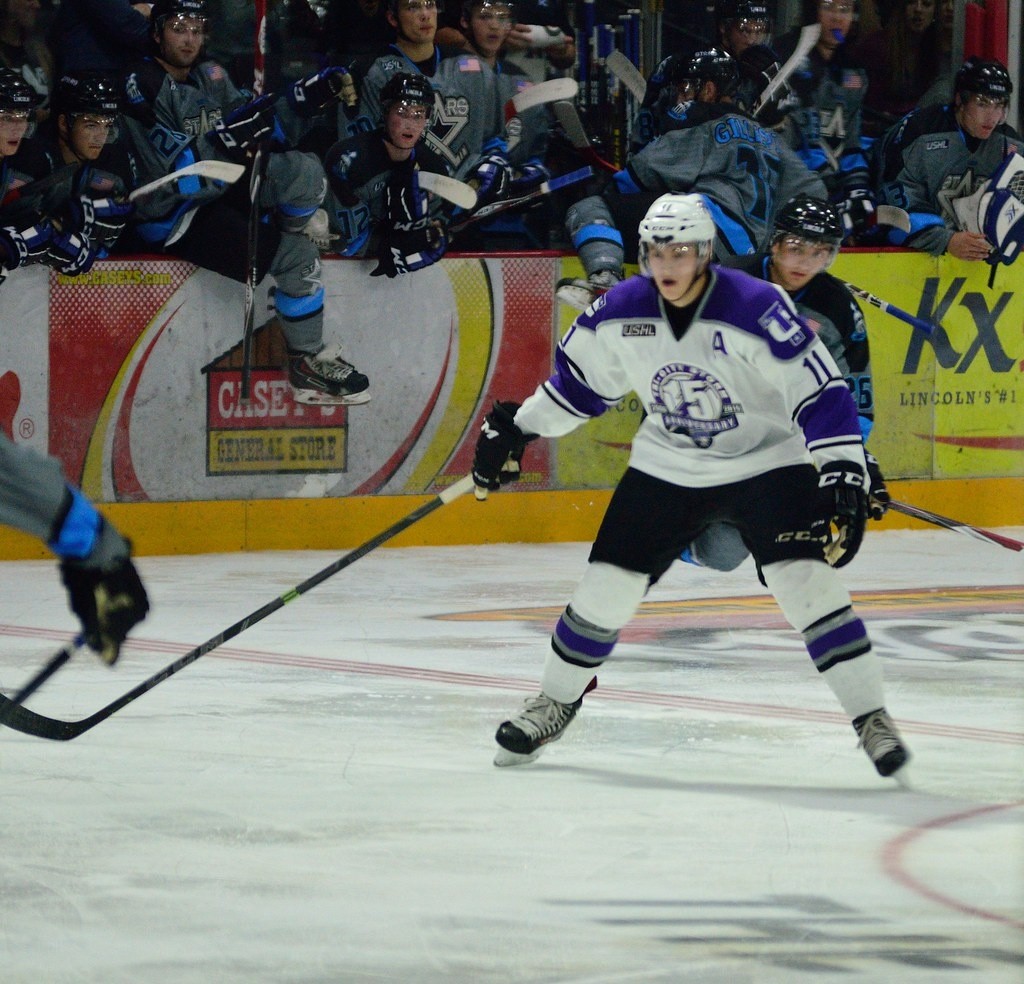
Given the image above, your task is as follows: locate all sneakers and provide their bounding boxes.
[287,339,371,406]
[492,677,598,768]
[853,706,913,788]
[283,221,333,255]
[553,266,627,312]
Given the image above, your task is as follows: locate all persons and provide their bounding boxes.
[676,194,892,572]
[0,0,1023,407]
[0,419,149,667]
[473,192,917,790]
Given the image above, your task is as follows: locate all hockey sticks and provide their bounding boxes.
[127,158,247,203]
[553,99,623,176]
[418,170,479,210]
[745,23,822,120]
[0,624,94,720]
[445,164,595,227]
[866,490,1022,554]
[504,77,578,131]
[830,270,938,339]
[605,49,648,113]
[0,453,517,746]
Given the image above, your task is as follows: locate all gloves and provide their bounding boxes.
[369,219,448,279]
[287,66,356,119]
[203,91,280,165]
[470,397,540,501]
[44,480,150,665]
[830,185,884,247]
[464,133,553,217]
[863,449,892,517]
[811,459,869,569]
[4,211,97,277]
[81,194,134,249]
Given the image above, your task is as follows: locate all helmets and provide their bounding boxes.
[381,0,521,119]
[681,0,862,103]
[0,68,47,137]
[770,192,844,275]
[147,0,211,43]
[954,56,1014,125]
[55,72,120,144]
[637,192,715,277]
